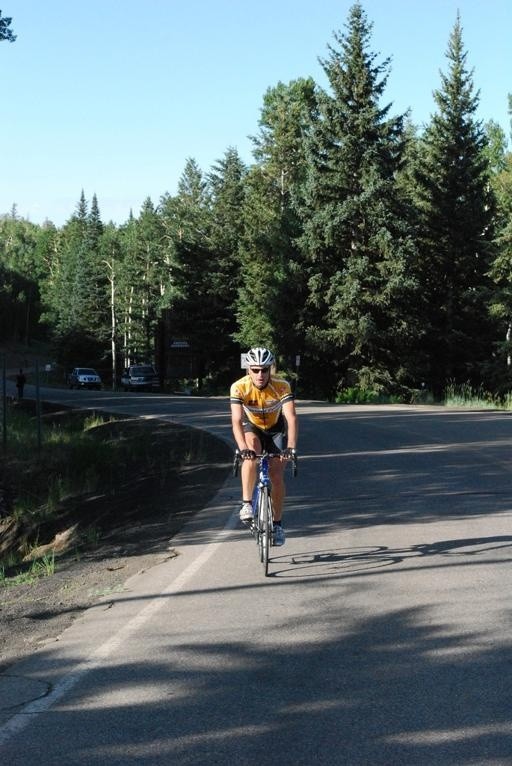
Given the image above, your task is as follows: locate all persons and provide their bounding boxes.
[229,346,299,546]
[15,368,27,399]
[113,367,131,393]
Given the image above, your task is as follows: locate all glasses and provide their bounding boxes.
[251,369,268,373]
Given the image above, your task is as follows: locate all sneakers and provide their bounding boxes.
[239,503,254,520]
[273,525,285,545]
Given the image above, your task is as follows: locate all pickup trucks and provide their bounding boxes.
[120,365,162,392]
[68,368,102,390]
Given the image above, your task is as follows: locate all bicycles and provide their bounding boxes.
[231,450,299,577]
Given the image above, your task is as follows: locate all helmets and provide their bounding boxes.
[244,347,274,366]
